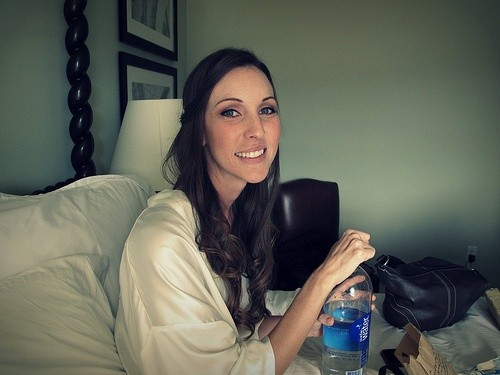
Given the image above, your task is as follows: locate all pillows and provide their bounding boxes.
[0,174,151,318]
[0,252,128,375]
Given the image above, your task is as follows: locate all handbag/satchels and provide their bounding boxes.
[374,253,490,332]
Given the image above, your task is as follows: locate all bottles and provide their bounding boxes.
[320,266,373,375]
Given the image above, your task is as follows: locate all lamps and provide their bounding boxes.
[108,98,185,192]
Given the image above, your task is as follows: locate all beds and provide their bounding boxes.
[0,174,500,375]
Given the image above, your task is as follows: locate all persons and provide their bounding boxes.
[114,47,376,375]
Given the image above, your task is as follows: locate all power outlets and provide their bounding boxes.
[465,245,478,265]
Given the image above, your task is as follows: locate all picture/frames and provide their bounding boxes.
[117,51,178,127]
[118,0,179,62]
